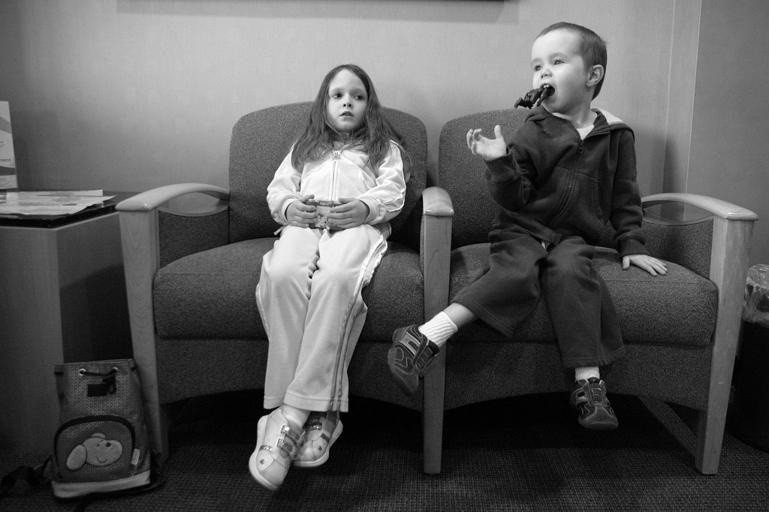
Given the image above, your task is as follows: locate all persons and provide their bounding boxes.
[386,20,668,431]
[247,63,408,493]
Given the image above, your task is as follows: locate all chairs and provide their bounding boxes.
[436,99,762,480]
[115,98,436,480]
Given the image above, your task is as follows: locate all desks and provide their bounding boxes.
[0,189,139,470]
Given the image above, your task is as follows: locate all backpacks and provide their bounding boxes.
[37,359,168,499]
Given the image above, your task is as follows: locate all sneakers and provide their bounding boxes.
[249,402,343,492]
[387,324,440,395]
[570,377,619,429]
